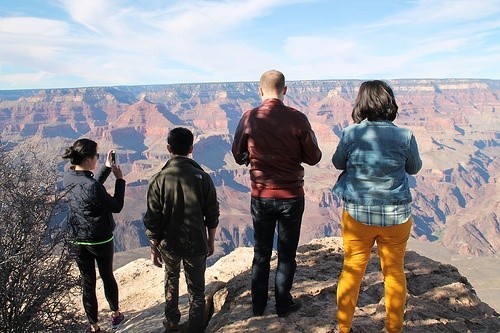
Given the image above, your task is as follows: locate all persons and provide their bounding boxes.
[142,127,220,333]
[61,139,126,333]
[232,70,322,318]
[332,80,422,333]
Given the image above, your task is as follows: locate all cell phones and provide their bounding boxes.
[111,150,116,166]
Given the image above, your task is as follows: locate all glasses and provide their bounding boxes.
[95,153,99,160]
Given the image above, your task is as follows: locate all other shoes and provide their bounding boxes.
[252,306,266,316]
[276,298,301,318]
[112,312,125,325]
[88,326,100,333]
[162,318,178,330]
